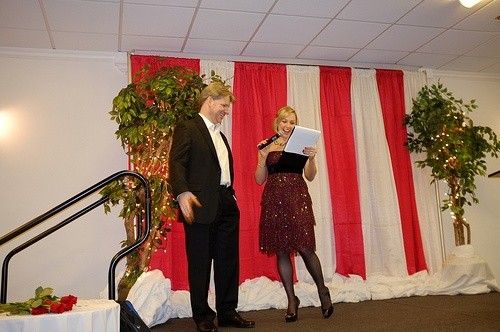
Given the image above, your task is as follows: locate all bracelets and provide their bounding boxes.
[256,160,268,169]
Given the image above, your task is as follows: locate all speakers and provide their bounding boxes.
[116,301,151,332]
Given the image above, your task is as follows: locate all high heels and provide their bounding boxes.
[285,296,300,322]
[319,287,334,318]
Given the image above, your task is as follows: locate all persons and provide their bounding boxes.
[168,80,256,332]
[254,106,335,321]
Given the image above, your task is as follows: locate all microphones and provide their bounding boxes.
[258,130,284,150]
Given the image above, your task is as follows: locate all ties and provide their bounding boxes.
[210,127,229,185]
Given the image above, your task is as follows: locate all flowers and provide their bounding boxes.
[0,287,78,315]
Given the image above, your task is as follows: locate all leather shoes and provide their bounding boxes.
[197,318,218,332]
[217,311,255,328]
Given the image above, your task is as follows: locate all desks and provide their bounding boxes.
[0,299,122,331]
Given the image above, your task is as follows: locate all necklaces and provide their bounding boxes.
[272,136,290,147]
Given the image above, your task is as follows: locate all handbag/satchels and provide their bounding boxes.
[116,301,150,332]
